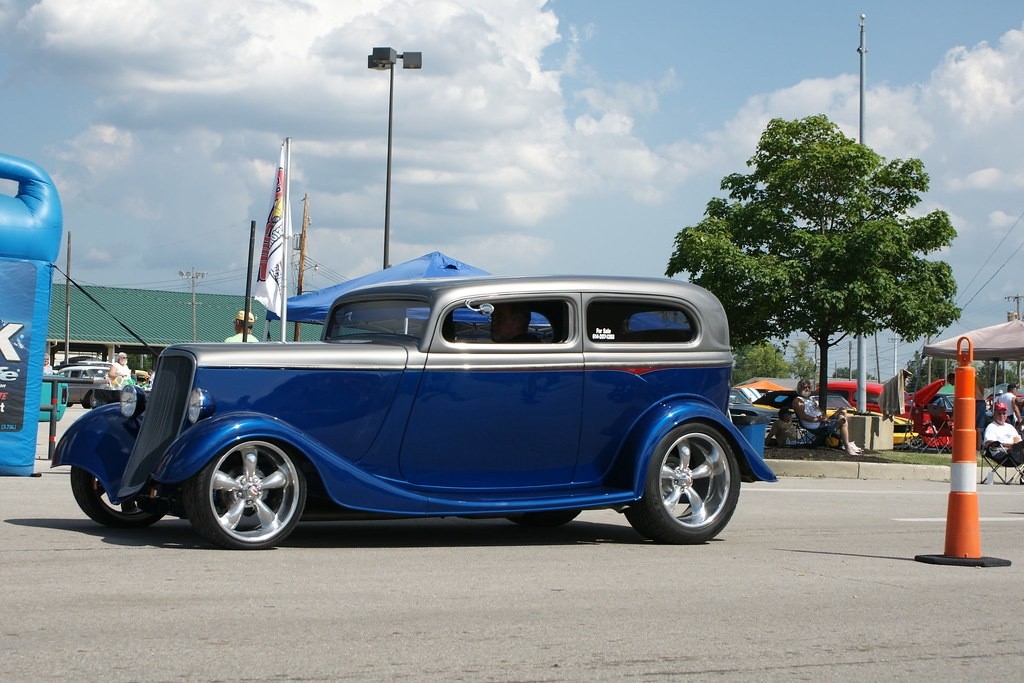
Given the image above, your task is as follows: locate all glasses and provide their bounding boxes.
[123,357,127,359]
[487,315,515,324]
[996,412,1006,415]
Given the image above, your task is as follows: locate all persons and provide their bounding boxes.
[997,383,1024,431]
[225,310,260,342]
[765,409,816,446]
[145,369,155,387]
[793,379,864,456]
[490,303,542,343]
[984,403,1024,484]
[947,373,986,451]
[43,353,53,374]
[108,352,130,384]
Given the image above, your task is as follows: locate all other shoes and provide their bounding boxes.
[841,441,864,453]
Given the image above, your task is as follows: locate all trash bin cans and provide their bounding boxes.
[731,415,770,461]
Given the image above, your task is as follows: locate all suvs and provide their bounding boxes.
[52,273,779,552]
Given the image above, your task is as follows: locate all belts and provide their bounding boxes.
[976,400,985,402]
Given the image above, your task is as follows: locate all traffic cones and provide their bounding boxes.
[912,334,1013,567]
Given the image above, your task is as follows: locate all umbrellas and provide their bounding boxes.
[753,391,799,409]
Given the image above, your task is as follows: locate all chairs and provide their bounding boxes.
[979,427,1024,485]
[793,408,841,449]
[902,405,954,455]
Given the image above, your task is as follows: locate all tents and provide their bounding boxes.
[736,381,793,391]
[263,251,551,340]
[904,320,1024,440]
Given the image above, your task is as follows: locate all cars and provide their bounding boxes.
[731,370,1024,452]
[54,353,156,411]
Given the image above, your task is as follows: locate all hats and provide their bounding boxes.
[1008,383,1020,389]
[778,408,791,415]
[994,402,1007,411]
[233,311,254,322]
[999,390,1003,394]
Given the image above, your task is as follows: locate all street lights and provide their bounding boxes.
[179,266,208,343]
[368,44,423,271]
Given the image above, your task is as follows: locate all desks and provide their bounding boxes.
[94,388,151,408]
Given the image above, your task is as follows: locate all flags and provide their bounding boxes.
[254,144,292,318]
